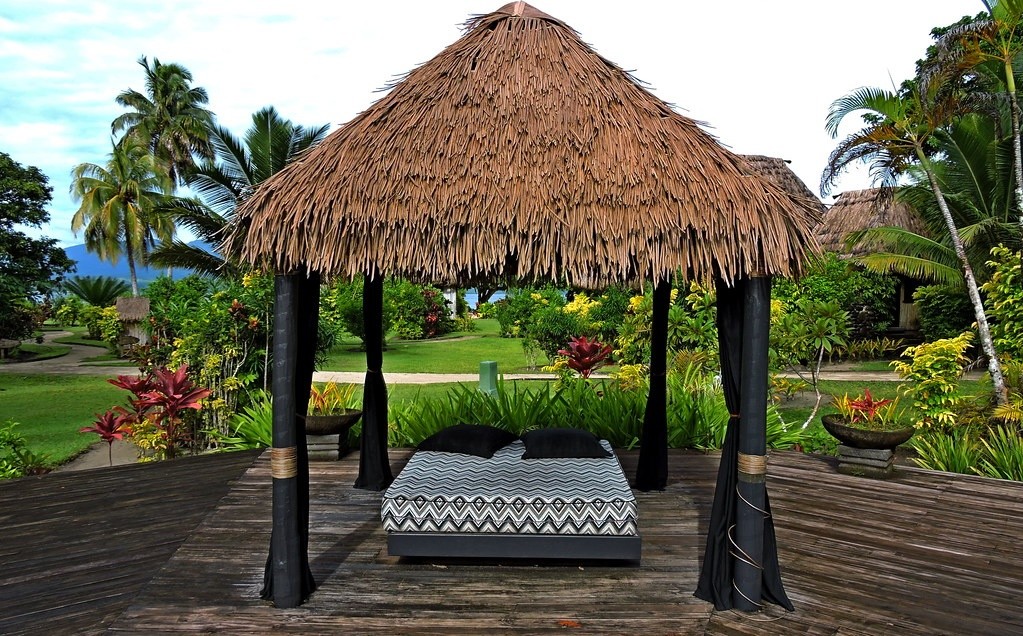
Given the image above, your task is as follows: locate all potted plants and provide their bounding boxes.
[821,388,917,447]
[305,375,363,435]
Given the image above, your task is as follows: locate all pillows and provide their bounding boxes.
[411,424,519,459]
[519,427,613,461]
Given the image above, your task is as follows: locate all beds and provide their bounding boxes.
[381,439,642,561]
